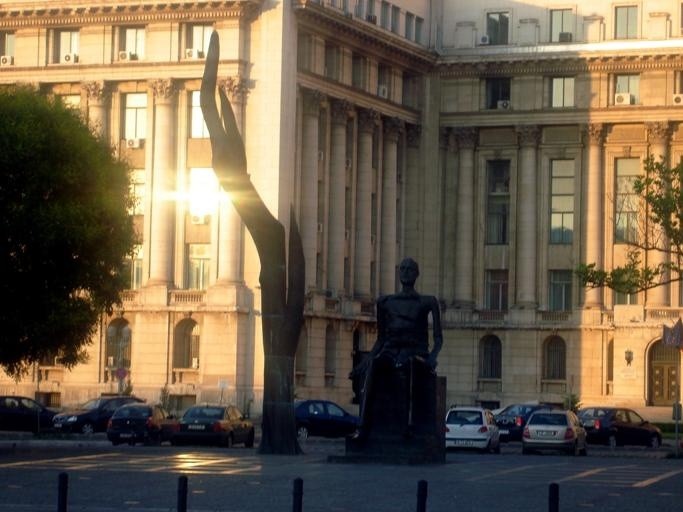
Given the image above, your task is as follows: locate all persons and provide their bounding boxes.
[354,259,443,444]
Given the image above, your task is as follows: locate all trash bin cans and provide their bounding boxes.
[31,407,50,440]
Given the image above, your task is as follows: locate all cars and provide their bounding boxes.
[110,402,178,447]
[578,407,664,454]
[294,399,360,439]
[524,408,587,457]
[174,402,255,449]
[0,395,58,432]
[492,402,561,443]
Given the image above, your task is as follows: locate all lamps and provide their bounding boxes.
[624,347,633,367]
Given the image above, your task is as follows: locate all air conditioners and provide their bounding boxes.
[119,51,131,62]
[479,35,489,46]
[671,93,683,106]
[0,54,14,67]
[614,92,633,105]
[496,100,511,111]
[377,84,388,99]
[315,150,376,249]
[63,53,77,64]
[184,48,199,60]
[125,137,140,149]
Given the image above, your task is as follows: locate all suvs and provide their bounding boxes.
[51,394,148,438]
[444,403,501,454]
